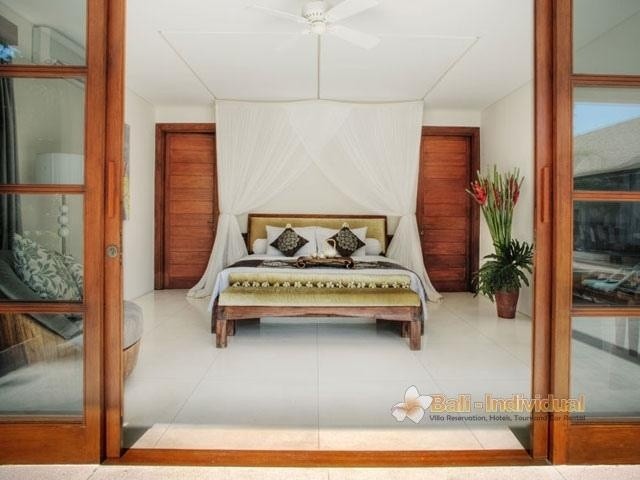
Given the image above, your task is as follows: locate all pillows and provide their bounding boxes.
[11,233,86,321]
[253,223,381,256]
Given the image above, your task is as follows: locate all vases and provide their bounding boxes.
[495,286,520,320]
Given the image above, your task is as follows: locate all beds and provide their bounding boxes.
[210,213,426,351]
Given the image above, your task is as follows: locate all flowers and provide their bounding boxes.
[462,166,534,298]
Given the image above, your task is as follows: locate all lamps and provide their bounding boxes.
[35,153,85,254]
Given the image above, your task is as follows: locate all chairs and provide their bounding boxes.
[0,251,141,383]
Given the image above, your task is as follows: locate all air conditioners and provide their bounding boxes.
[32,27,86,87]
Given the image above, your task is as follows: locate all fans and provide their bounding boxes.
[241,1,382,51]
